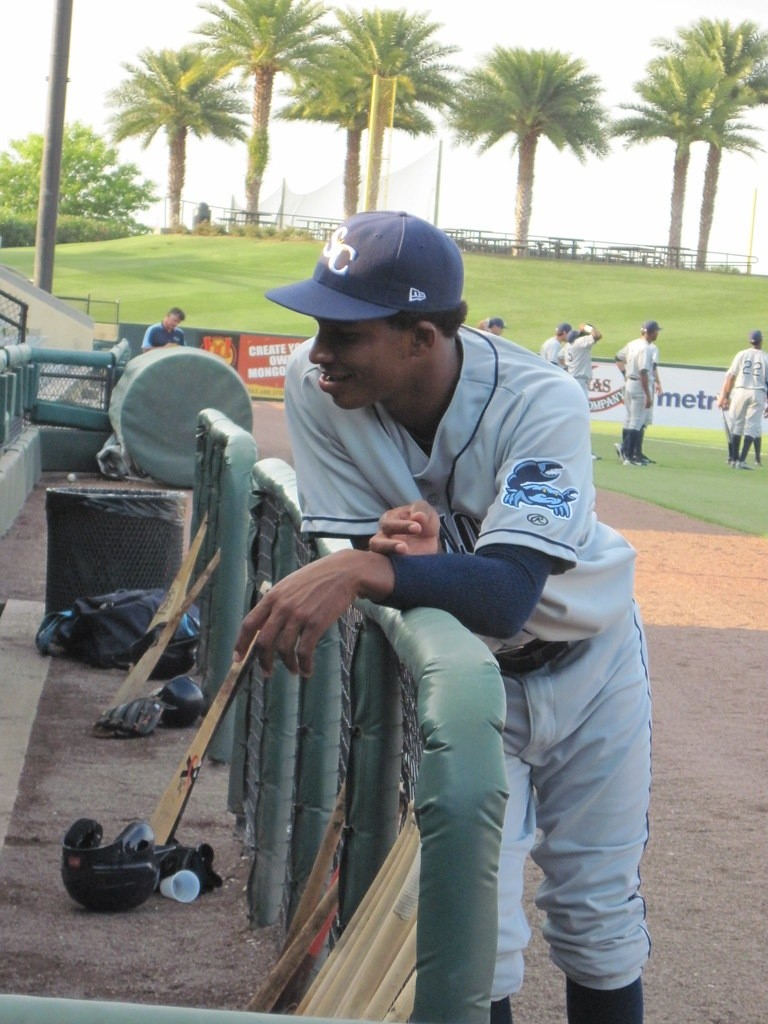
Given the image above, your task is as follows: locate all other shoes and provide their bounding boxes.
[592,453,601,460]
[731,461,736,466]
[727,457,731,463]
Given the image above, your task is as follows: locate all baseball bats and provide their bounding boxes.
[128,512,208,674]
[245,778,423,1024]
[107,550,221,712]
[148,581,274,846]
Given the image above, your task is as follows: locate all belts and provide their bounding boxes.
[493,638,566,674]
[629,377,637,380]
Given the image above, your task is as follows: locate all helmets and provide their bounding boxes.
[156,674,203,727]
[62,815,177,912]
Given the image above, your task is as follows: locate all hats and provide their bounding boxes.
[567,331,582,342]
[749,330,761,342]
[264,210,464,321]
[488,317,508,329]
[556,323,571,332]
[644,320,663,332]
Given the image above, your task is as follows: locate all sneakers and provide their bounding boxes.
[756,463,764,466]
[615,443,625,462]
[623,456,648,466]
[736,461,753,470]
[633,453,656,464]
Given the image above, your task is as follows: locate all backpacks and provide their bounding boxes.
[36,588,200,679]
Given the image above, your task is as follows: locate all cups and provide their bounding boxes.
[160,869,201,903]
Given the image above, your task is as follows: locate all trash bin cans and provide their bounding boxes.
[44,486,188,617]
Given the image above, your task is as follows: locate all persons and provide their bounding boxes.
[235,214,653,1024]
[718,330,768,469]
[478,316,508,336]
[614,321,663,467]
[541,322,603,461]
[140,307,186,352]
